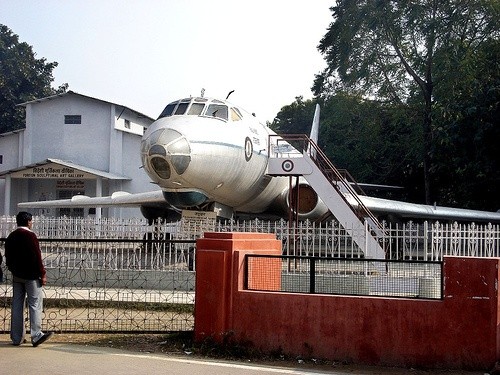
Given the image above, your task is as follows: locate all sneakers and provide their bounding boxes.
[13,342,20,346]
[32,332,54,347]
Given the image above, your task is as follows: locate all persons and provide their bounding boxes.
[4,211,54,348]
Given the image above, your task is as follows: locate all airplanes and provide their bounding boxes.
[17,89,500,271]
